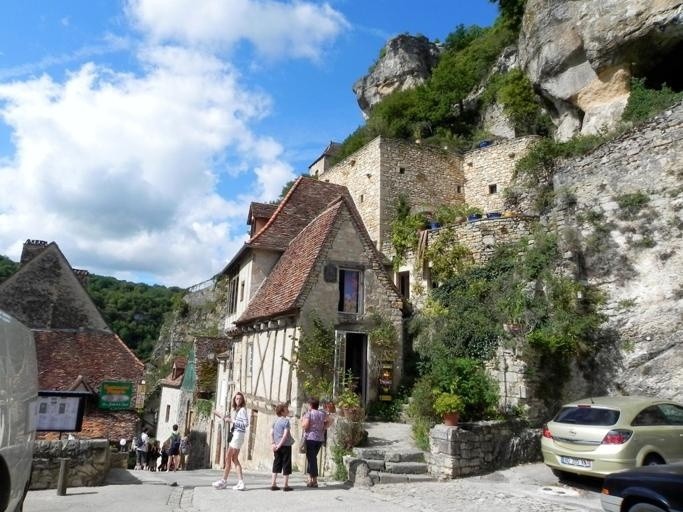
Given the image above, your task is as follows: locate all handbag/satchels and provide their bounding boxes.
[298,438,307,453]
[228,432,232,442]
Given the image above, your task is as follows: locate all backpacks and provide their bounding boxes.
[133,432,146,447]
[172,432,180,449]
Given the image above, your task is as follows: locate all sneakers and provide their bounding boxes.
[213,480,226,488]
[283,487,292,490]
[237,481,244,489]
[135,466,155,471]
[307,482,317,487]
[272,486,279,490]
[159,467,178,471]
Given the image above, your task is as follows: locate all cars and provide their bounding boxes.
[0,307,39,512]
[540,396,682,484]
[600,463,682,512]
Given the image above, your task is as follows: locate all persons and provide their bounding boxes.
[300,395,335,489]
[116,424,193,472]
[270,402,295,492]
[210,392,249,490]
